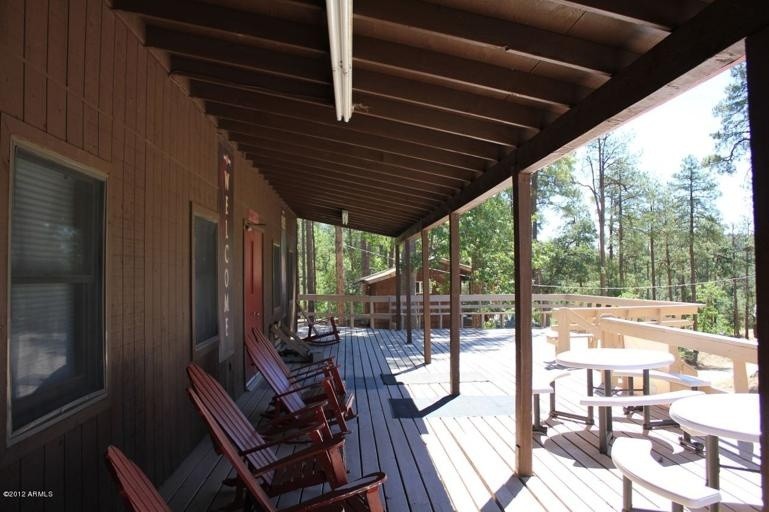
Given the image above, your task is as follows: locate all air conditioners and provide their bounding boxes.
[416,280,433,296]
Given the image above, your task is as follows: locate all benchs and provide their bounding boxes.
[531,367,570,433]
[580,388,705,454]
[614,369,710,452]
[611,436,722,512]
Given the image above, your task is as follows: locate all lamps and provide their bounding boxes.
[341,210,348,225]
[327,0,353,123]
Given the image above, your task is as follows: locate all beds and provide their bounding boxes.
[555,348,675,438]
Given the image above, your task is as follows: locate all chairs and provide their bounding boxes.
[297,302,340,346]
[187,362,349,512]
[188,388,387,512]
[245,335,359,444]
[108,447,169,512]
[253,328,346,407]
